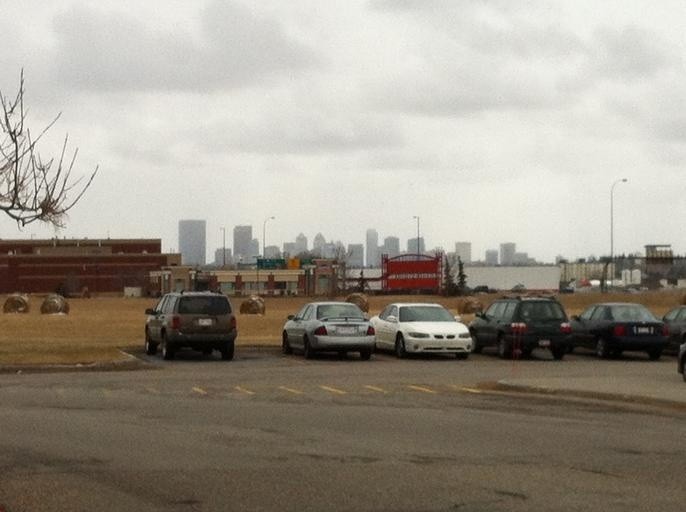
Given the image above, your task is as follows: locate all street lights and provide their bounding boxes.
[611,178,628,292]
[220,227,225,265]
[263,216,276,268]
[413,215,420,254]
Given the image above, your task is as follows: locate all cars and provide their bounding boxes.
[145,289,238,360]
[563,287,573,293]
